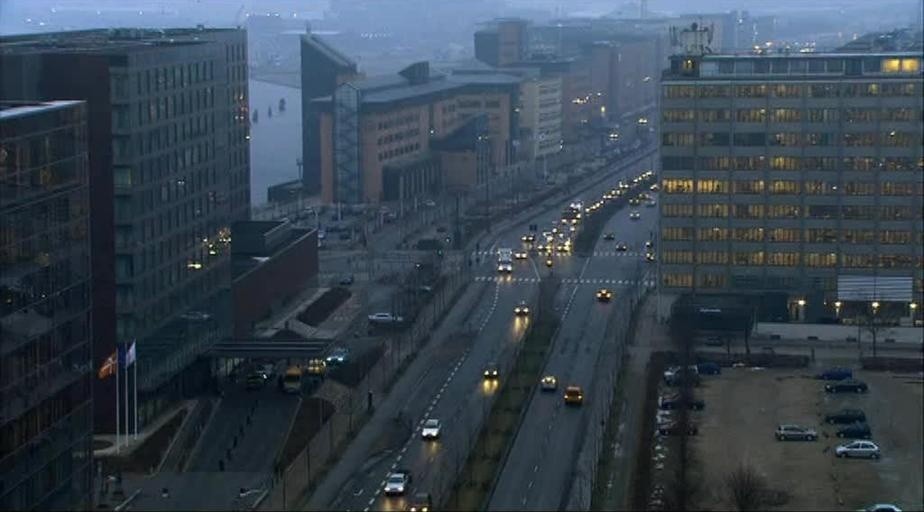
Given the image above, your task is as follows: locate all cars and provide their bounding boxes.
[338,271,355,285]
[609,130,619,139]
[421,418,441,441]
[835,420,872,441]
[368,312,404,324]
[638,116,648,124]
[822,376,868,395]
[407,491,432,512]
[383,473,409,497]
[277,200,437,248]
[647,354,721,512]
[513,299,531,316]
[397,283,432,295]
[563,384,585,406]
[855,503,904,512]
[816,366,856,381]
[595,286,613,302]
[281,365,303,395]
[307,358,327,377]
[705,336,723,347]
[482,359,501,378]
[326,347,350,366]
[825,407,868,426]
[834,439,884,460]
[540,374,558,391]
[495,167,657,273]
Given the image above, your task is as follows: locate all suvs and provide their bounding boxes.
[775,423,817,441]
